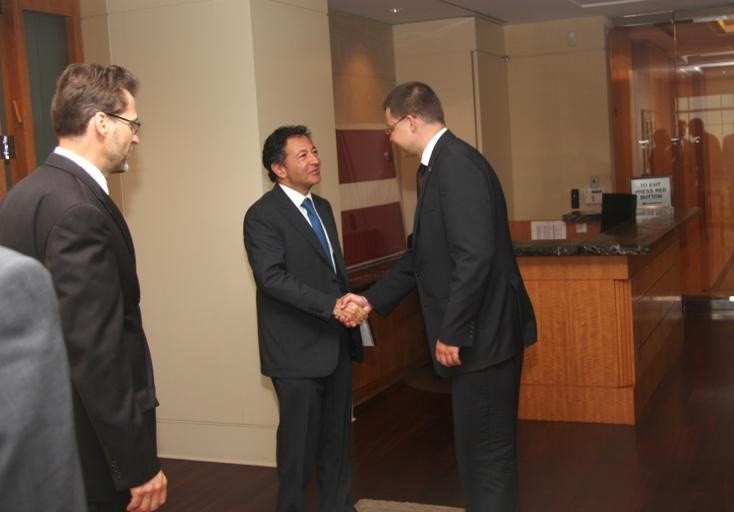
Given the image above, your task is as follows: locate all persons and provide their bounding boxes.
[687,118,721,159]
[339,82,539,511]
[243,124,366,510]
[649,128,676,178]
[0,59,171,512]
[0,241,91,511]
[673,118,691,159]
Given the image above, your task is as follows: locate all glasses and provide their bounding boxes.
[107,111,143,136]
[384,114,415,138]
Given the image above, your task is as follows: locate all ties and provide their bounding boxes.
[415,163,428,199]
[301,197,333,274]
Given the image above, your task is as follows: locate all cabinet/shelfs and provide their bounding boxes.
[341,252,432,423]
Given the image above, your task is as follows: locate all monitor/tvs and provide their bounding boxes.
[601,193,636,232]
